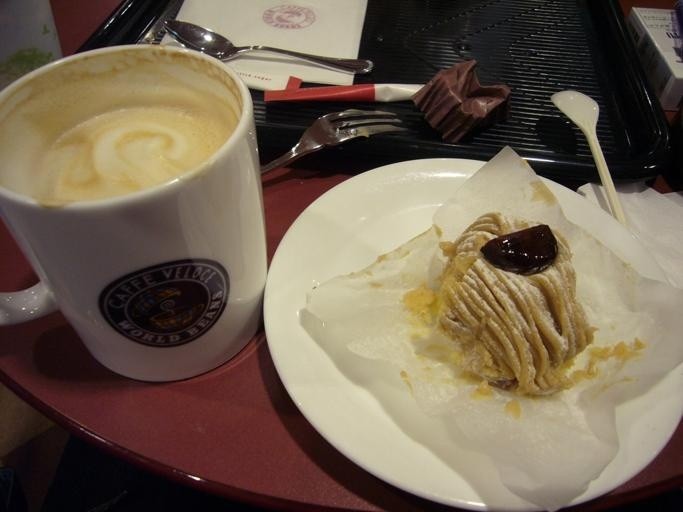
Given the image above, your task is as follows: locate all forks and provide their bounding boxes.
[253,101,405,180]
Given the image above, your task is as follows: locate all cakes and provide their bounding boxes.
[403,210,600,398]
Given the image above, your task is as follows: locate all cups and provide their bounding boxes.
[0,45,268,383]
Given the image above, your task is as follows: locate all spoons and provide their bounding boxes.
[164,17,375,74]
[551,89,626,227]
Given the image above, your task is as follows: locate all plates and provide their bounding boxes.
[262,153,682,512]
